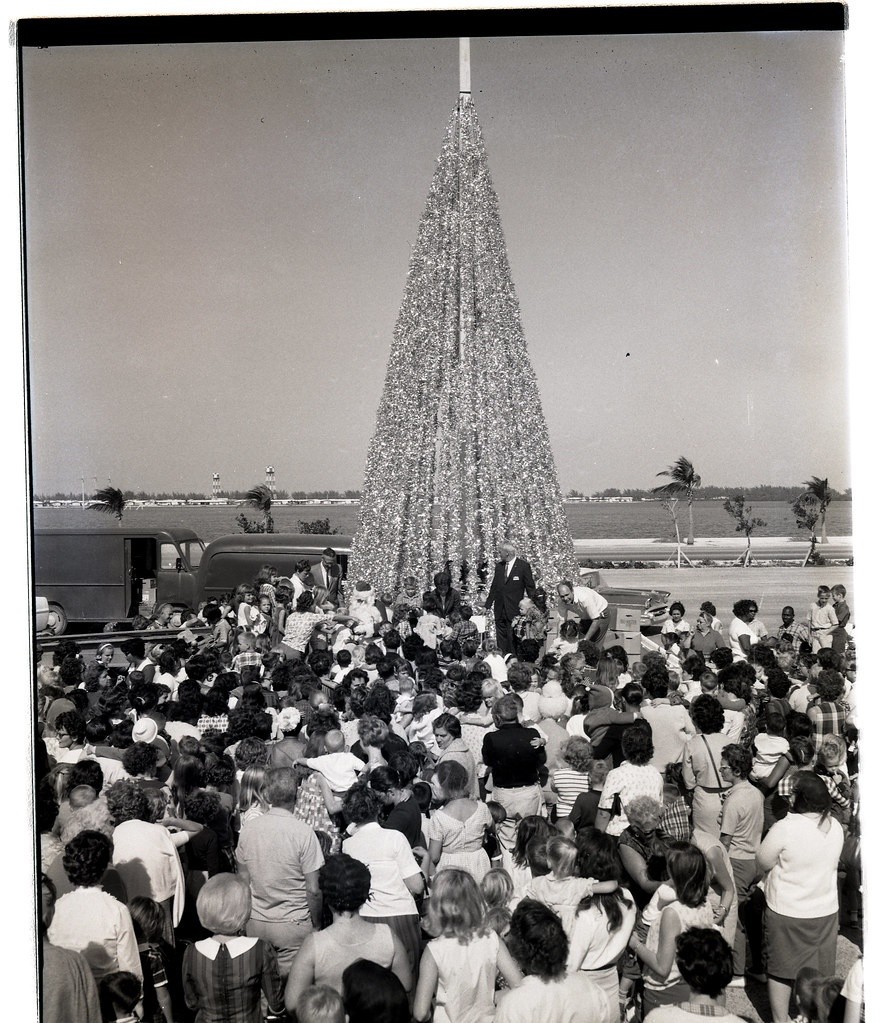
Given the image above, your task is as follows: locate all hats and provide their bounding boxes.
[130,717,157,744]
[278,707,300,732]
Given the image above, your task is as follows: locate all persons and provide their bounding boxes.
[38,546,863,1023]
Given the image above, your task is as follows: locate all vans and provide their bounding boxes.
[35,526,207,636]
[191,535,353,618]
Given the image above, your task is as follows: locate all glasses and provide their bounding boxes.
[717,763,731,770]
[55,731,68,737]
[574,665,585,670]
[695,620,705,624]
[749,608,757,612]
[321,557,335,566]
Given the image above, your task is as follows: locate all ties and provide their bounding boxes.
[503,562,508,582]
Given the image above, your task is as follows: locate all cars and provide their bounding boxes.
[578,567,670,630]
